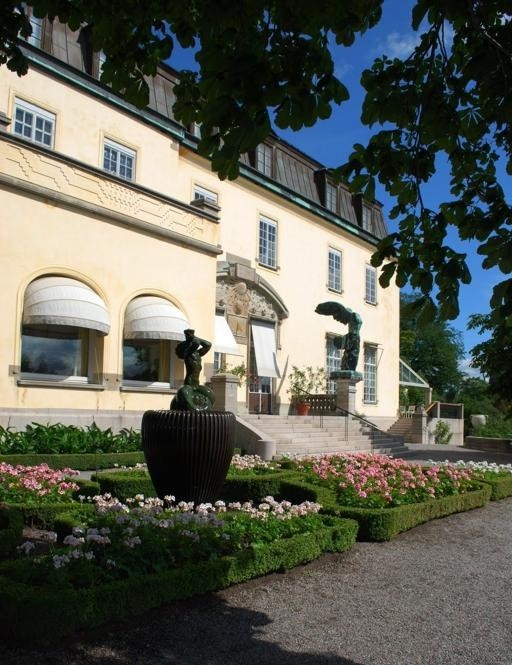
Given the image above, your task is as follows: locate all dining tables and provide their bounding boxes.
[286,365,325,415]
